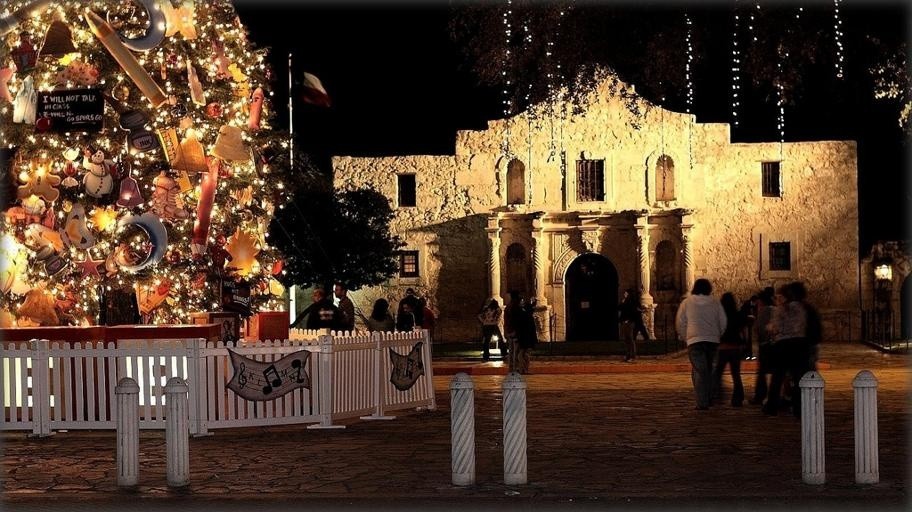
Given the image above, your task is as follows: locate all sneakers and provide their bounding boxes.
[624,356,638,363]
[693,391,801,420]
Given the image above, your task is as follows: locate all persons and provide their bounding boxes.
[675,278,822,416]
[637,287,652,341]
[289,282,435,331]
[476,290,536,375]
[617,288,638,364]
